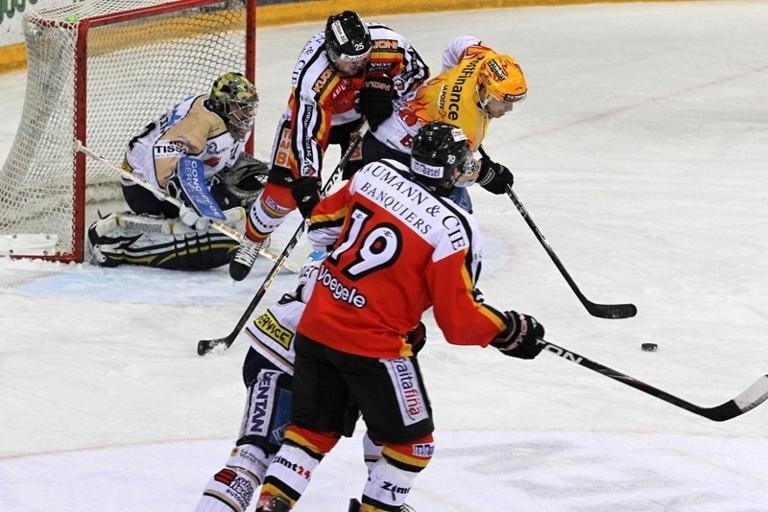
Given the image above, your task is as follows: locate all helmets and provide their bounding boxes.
[324,10,372,70]
[409,121,471,185]
[211,72,259,143]
[476,54,528,105]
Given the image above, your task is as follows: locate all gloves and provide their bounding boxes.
[475,161,513,194]
[489,310,544,359]
[291,182,320,218]
[359,77,394,132]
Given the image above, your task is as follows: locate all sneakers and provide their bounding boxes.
[229,231,263,281]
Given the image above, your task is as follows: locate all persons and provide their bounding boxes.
[191,244,428,511]
[227,8,431,282]
[359,32,527,216]
[255,118,548,512]
[84,70,270,273]
